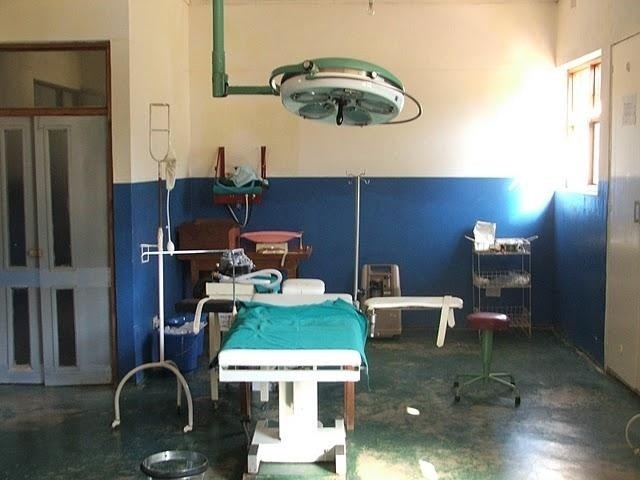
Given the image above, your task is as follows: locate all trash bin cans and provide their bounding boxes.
[153,312,206,375]
[140,451,209,479]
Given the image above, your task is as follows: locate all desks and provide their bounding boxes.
[175,244,313,297]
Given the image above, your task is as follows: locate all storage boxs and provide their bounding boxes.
[177,216,241,250]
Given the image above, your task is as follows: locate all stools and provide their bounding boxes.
[454,311,523,407]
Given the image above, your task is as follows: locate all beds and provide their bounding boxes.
[215,278,362,476]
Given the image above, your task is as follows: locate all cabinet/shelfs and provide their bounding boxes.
[470,237,533,343]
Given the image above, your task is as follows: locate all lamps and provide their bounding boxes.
[209,0,421,129]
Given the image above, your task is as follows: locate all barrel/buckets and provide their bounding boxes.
[168,312,209,357]
[158,323,201,375]
